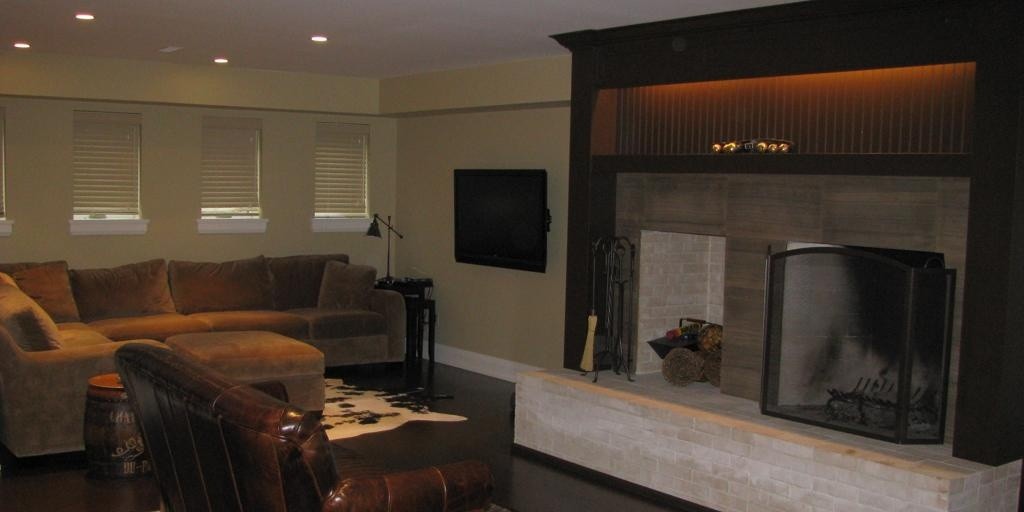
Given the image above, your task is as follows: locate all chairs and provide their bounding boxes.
[114,338,496,511]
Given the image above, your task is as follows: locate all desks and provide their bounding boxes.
[378,283,435,361]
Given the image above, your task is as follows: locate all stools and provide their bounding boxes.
[163,329,326,418]
[82,371,153,483]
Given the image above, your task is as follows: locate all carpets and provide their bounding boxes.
[320,377,469,441]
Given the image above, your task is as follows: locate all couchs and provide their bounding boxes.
[0,289,408,476]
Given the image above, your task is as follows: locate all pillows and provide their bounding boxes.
[319,261,376,311]
[70,259,174,319]
[168,254,270,316]
[0,275,59,351]
[13,260,80,322]
[266,254,348,314]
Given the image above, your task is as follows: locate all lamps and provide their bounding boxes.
[366,213,403,283]
